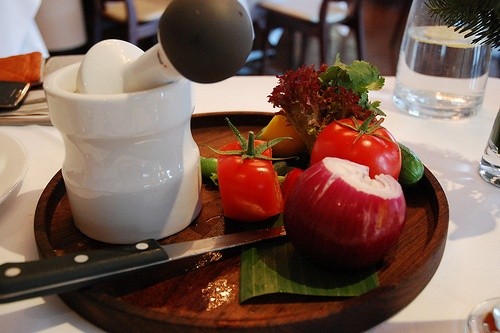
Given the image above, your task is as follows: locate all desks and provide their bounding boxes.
[0,75,500,332]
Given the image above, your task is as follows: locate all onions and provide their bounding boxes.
[284,157,406,272]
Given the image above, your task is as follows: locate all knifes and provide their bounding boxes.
[0,226,286,305]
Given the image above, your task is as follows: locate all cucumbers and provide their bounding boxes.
[398,142,424,182]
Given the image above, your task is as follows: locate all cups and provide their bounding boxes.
[43,59,203,246]
[479,108,500,187]
[393,1,493,120]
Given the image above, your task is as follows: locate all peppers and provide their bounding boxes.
[201,110,303,184]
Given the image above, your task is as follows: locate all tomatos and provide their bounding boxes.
[310,118,402,179]
[217,140,283,221]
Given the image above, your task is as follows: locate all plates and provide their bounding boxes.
[0,129,30,208]
[464,297,500,333]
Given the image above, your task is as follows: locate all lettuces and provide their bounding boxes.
[269,55,385,133]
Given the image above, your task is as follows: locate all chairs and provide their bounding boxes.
[89,0,170,52]
[256,0,366,77]
